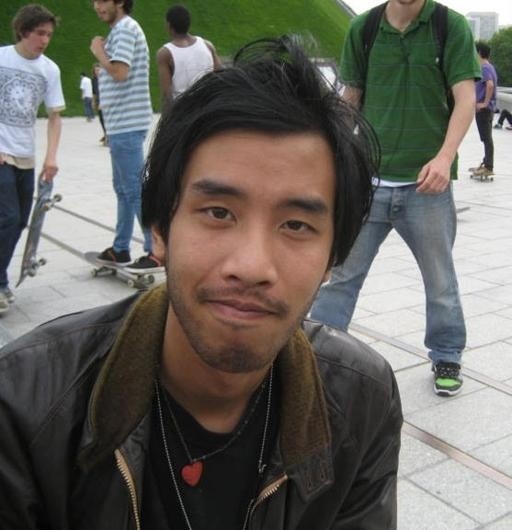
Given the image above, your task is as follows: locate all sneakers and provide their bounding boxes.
[493,125,502,128]
[505,126,512,130]
[473,166,493,175]
[0,289,16,313]
[96,246,132,267]
[433,362,464,395]
[124,254,166,274]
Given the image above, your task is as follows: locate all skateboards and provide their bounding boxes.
[85,252,167,289]
[15,170,62,288]
[469,166,495,182]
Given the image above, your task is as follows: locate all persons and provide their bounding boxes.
[473,38,496,178]
[305,0,479,399]
[92,63,107,143]
[156,4,226,119]
[1,36,404,530]
[1,2,67,314]
[88,0,168,273]
[80,72,95,122]
[495,107,511,129]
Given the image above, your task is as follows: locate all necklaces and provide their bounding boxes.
[147,364,271,529]
[155,366,271,488]
